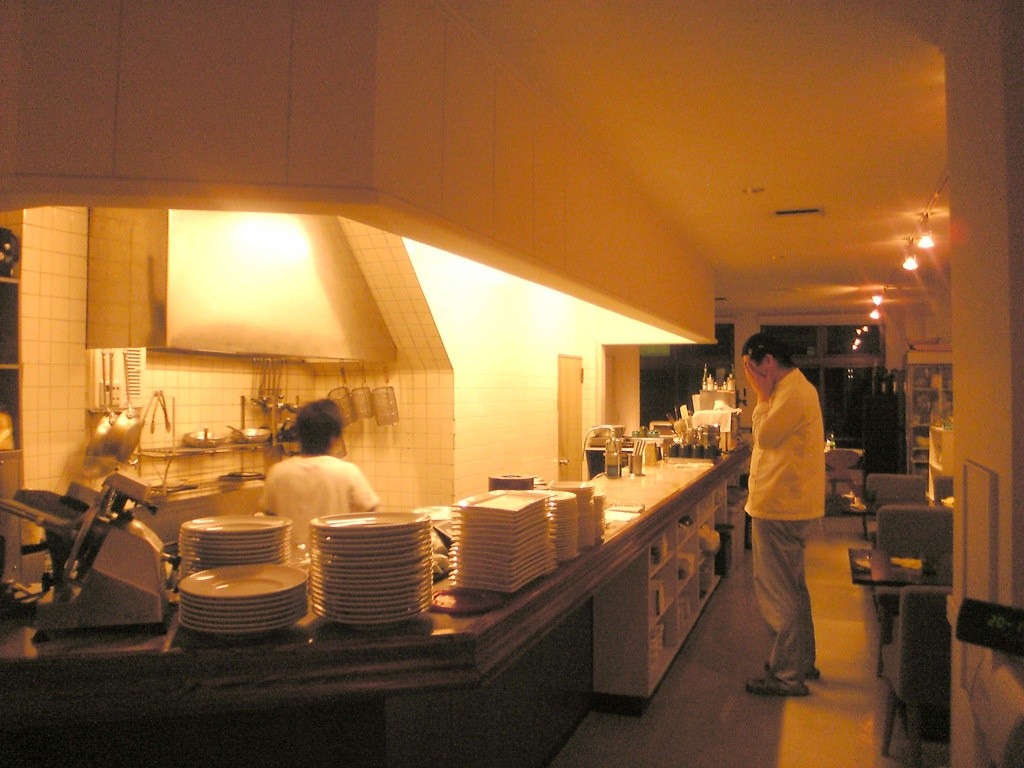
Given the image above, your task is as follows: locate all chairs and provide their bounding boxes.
[826,448,864,500]
[864,473,954,768]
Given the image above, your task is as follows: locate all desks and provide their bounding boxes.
[584,446,634,481]
[842,494,946,543]
[822,447,866,458]
[847,547,954,587]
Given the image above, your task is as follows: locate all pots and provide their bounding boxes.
[83,350,270,461]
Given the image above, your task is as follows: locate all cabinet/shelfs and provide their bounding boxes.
[904,350,953,504]
[592,478,730,700]
[0,279,24,503]
[137,440,288,502]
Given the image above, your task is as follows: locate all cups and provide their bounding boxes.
[628,455,642,475]
[669,444,715,458]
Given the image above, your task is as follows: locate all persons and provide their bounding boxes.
[261,399,379,571]
[742,332,826,696]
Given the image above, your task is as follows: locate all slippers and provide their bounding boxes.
[765,661,821,678]
[746,672,809,697]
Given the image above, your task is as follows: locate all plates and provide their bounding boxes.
[447,480,615,594]
[307,511,434,632]
[177,515,308,636]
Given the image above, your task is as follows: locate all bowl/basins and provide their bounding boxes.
[592,425,626,437]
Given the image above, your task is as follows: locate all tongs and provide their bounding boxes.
[141,391,169,433]
[151,391,170,434]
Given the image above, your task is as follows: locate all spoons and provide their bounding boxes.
[251,356,303,414]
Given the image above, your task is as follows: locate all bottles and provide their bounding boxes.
[702,367,734,391]
